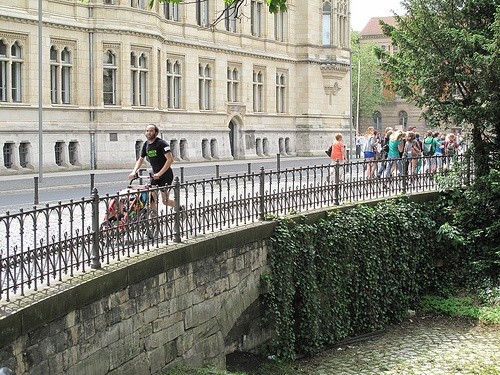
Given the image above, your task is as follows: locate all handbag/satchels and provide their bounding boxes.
[373,147,379,157]
[325,145,332,157]
[411,145,420,157]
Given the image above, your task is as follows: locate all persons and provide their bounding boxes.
[128,124,186,229]
[355,127,464,189]
[330,133,346,184]
[103,201,132,227]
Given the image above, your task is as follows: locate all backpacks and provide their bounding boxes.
[424,139,434,156]
[447,141,455,154]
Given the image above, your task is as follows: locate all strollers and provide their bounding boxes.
[99,172,159,246]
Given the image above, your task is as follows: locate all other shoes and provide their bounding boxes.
[180,205,185,222]
